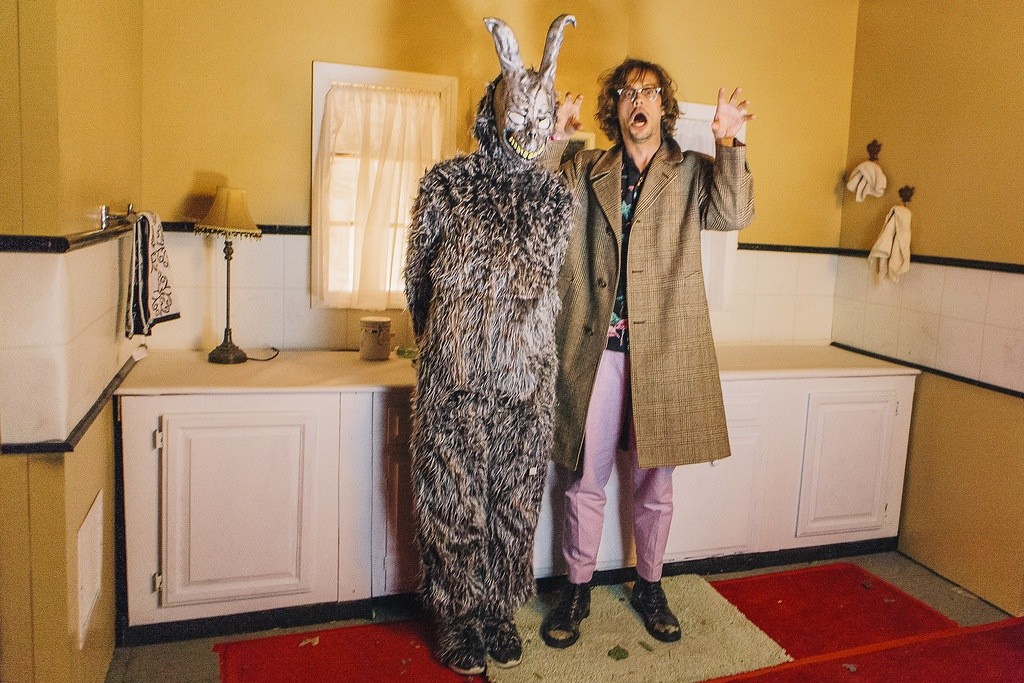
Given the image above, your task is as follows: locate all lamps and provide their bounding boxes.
[191,184,263,366]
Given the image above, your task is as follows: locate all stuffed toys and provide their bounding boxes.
[400,13,579,674]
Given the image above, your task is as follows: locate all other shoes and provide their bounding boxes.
[451,657,485,674]
[487,646,522,668]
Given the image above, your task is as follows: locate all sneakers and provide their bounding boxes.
[630,577,681,642]
[542,583,591,648]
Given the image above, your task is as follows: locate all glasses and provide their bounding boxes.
[617,87,661,102]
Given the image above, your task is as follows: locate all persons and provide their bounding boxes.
[535,55,755,650]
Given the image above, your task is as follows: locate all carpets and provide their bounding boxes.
[212,561,1024,683]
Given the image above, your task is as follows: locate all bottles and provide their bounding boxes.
[360,316,391,360]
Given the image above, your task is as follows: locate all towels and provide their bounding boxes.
[867,205,912,288]
[846,160,887,202]
[124,209,181,340]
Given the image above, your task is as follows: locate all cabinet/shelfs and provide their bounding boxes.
[117,374,920,646]
